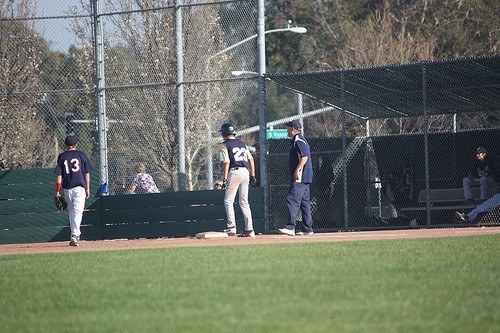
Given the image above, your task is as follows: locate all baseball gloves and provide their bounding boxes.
[54,194,67,212]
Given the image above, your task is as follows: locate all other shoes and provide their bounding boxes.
[454,211,470,224]
[463,199,486,204]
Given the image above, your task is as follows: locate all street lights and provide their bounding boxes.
[231,70,305,141]
[206,26,307,190]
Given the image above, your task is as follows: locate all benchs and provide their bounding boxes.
[418,185,500,209]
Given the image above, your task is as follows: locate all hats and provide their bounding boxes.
[221,123,236,136]
[64,135,79,146]
[475,147,488,154]
[285,120,301,131]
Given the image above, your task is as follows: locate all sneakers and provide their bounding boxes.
[278,227,295,236]
[296,232,314,235]
[237,231,256,237]
[221,228,237,235]
[69,236,79,247]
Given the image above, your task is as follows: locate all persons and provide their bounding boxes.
[125,164,161,195]
[278,120,314,237]
[451,193,500,227]
[111,179,128,196]
[390,145,421,227]
[55,136,92,245]
[460,147,500,205]
[214,180,225,190]
[311,147,335,230]
[219,124,256,238]
